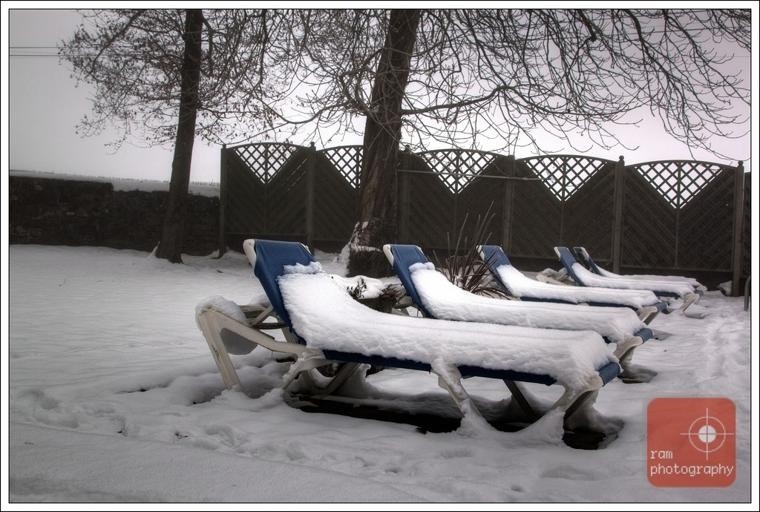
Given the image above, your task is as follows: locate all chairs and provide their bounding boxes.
[570,246,707,306]
[471,245,673,341]
[359,243,658,383]
[197,238,625,449]
[536,247,709,319]
